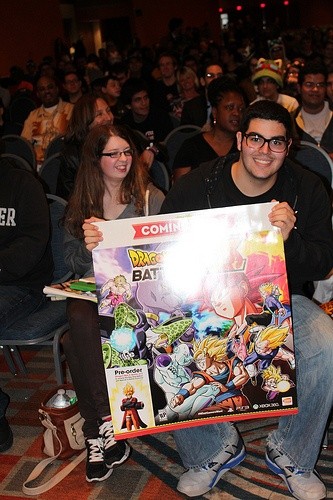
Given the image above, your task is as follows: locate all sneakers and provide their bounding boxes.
[265,443,327,500]
[96,417,133,469]
[81,419,113,484]
[176,424,246,497]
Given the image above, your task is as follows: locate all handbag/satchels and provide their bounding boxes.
[38,385,88,461]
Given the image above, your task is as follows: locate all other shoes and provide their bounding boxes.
[0,392,14,453]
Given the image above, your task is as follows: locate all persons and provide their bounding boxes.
[82,98,333,500]
[0,168,49,452]
[0,15,333,310]
[62,128,166,483]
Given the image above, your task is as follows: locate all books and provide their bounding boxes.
[43,277,97,302]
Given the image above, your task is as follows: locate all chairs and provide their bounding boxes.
[0,125,333,450]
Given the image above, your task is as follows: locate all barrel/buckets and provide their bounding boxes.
[40,383,87,458]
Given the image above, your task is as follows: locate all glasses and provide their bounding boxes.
[242,132,288,153]
[302,82,328,90]
[100,149,133,158]
[272,48,281,52]
[64,78,78,85]
[205,73,222,78]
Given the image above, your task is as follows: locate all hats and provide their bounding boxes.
[267,38,286,60]
[250,58,284,88]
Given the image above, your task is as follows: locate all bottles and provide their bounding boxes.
[54,389,70,407]
